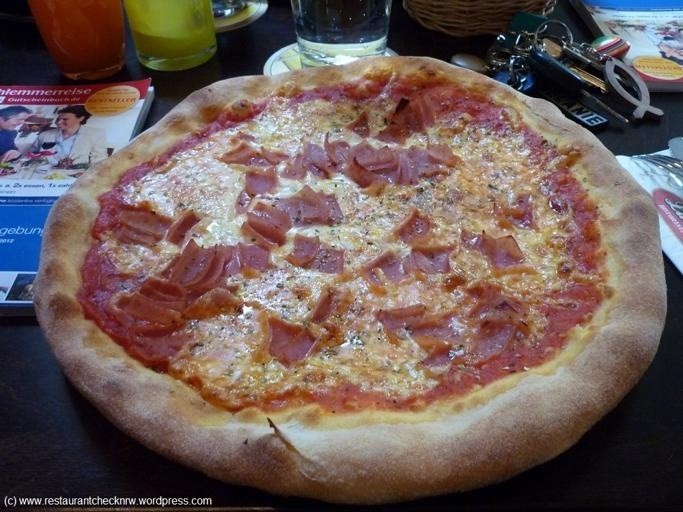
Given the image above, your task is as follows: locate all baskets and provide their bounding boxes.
[403,0,556,37]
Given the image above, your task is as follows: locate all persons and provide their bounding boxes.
[24,104,110,168]
[0,105,32,164]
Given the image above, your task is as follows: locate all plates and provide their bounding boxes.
[212,1,273,33]
[261,40,400,76]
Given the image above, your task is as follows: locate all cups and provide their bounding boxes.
[123,1,217,72]
[27,1,128,80]
[289,0,395,69]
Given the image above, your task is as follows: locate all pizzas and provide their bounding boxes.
[33,55,669,505]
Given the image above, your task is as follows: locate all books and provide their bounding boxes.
[566,1,682,94]
[0,77,155,320]
